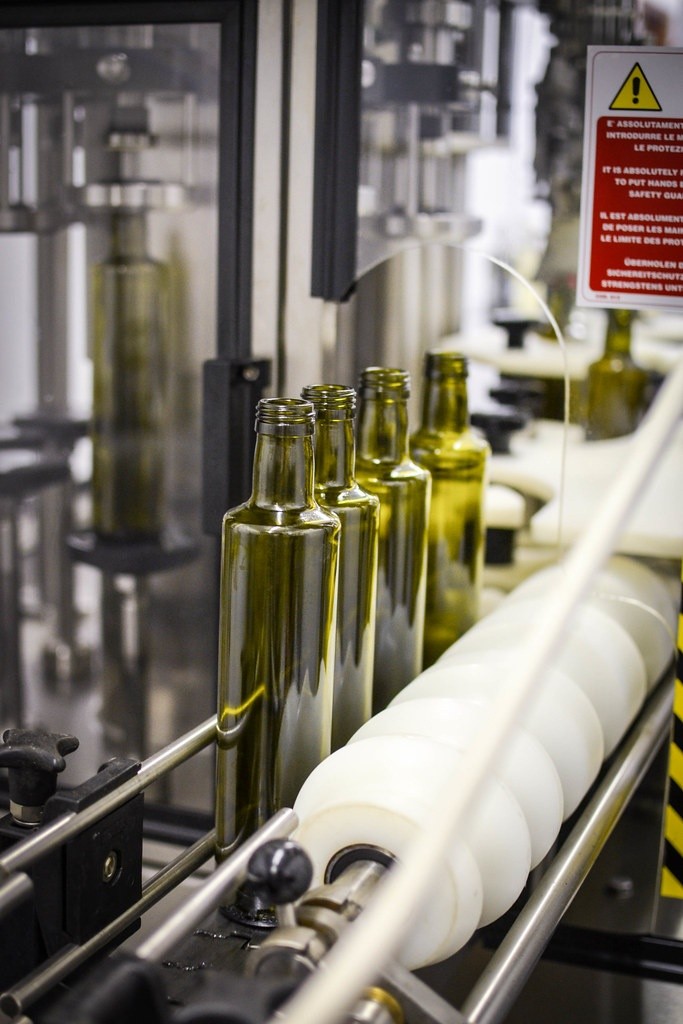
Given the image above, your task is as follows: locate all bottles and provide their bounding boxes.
[218,388,340,865]
[580,308,644,439]
[291,377,379,753]
[407,348,488,666]
[92,208,175,546]
[353,365,433,717]
